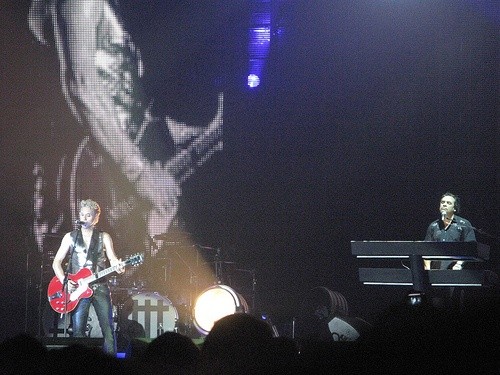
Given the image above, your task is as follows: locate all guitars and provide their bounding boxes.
[47,253,144,314]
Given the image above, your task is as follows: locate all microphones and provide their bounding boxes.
[75,220,87,226]
[441,211,447,221]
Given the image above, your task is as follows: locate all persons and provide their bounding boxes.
[52,200,125,354]
[28,0,220,339]
[422,193,476,270]
[0,291,500,375]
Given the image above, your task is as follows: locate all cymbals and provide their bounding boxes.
[201,261,255,269]
[153,232,193,241]
[177,245,216,251]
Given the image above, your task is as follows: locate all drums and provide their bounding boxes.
[119,292,186,339]
[151,257,174,285]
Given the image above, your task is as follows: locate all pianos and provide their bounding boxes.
[358,267,499,287]
[351,240,491,263]
